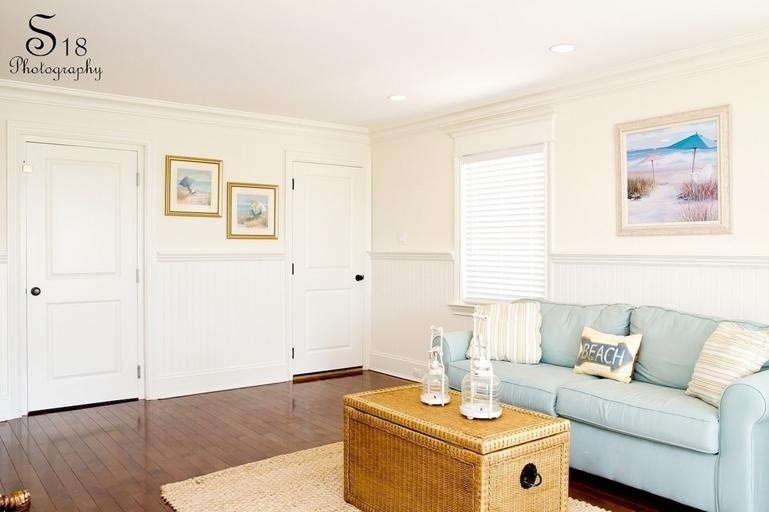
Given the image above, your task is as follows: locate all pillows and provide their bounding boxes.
[509,297,638,370]
[685,322,769,410]
[574,326,643,384]
[465,302,542,364]
[629,304,769,391]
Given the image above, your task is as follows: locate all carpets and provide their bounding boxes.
[160,442,610,512]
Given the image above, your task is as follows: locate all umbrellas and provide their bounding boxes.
[180,175,196,194]
[636,152,667,182]
[667,130,717,177]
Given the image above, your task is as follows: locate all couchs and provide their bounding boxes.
[433,328,769,512]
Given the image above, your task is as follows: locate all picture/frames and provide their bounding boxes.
[164,154,224,217]
[227,182,278,240]
[614,105,733,237]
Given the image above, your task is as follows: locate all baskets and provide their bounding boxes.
[341,403,570,511]
